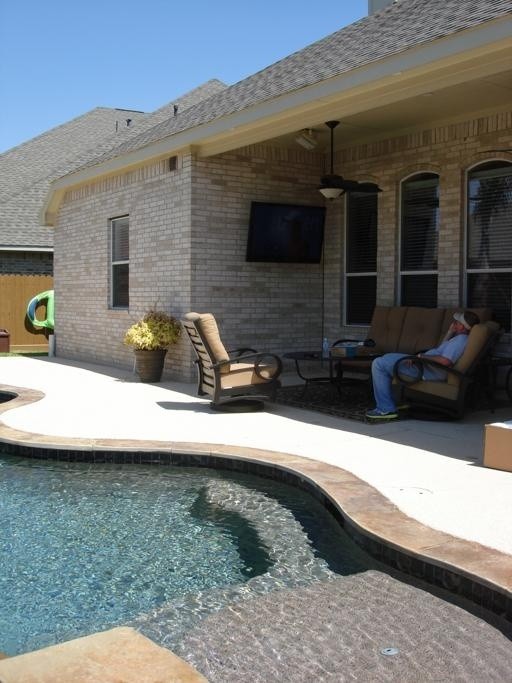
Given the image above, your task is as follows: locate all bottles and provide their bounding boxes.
[322,337,330,359]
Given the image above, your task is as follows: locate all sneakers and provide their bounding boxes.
[365,409,398,418]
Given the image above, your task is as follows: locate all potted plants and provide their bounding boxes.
[120,307,182,381]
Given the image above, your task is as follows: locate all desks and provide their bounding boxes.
[283,350,384,404]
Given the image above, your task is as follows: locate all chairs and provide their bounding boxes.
[329,305,499,423]
[176,310,284,410]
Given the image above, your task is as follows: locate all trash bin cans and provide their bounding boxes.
[0,329,10,353]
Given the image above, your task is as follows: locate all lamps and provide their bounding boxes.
[317,119,345,200]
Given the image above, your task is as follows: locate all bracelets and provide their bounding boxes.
[417,352,421,357]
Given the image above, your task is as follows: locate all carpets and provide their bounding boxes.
[269,378,406,425]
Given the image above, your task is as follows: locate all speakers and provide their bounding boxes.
[296,134,318,152]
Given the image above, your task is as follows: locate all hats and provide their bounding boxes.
[453,311,472,330]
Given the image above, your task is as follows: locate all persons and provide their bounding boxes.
[364,309,480,419]
